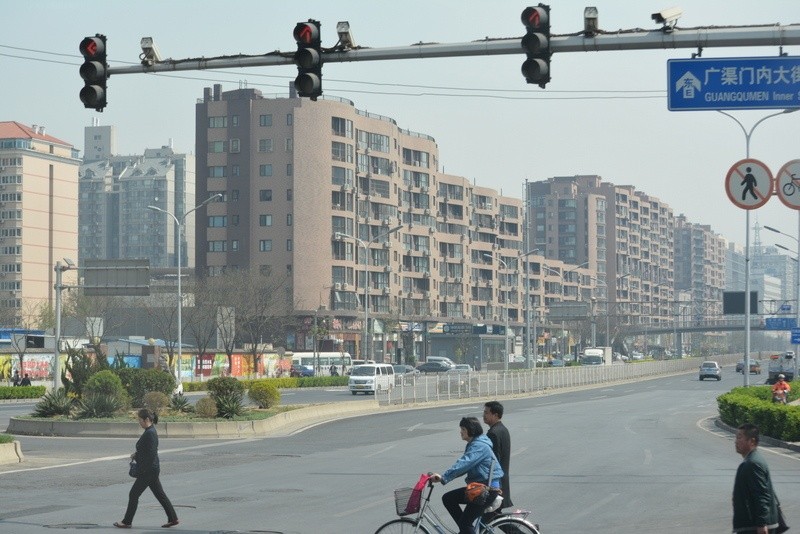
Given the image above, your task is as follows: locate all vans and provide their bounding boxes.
[348,363,394,395]
[291,351,352,376]
[768,353,795,382]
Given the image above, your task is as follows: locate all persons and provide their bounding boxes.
[732,423,780,534]
[329,363,337,376]
[483,401,525,534]
[771,374,791,405]
[290,365,299,377]
[113,408,179,528]
[19,373,31,387]
[429,417,505,534]
[13,370,20,387]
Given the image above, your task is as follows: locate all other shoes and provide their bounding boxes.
[114,522,132,528]
[162,519,178,527]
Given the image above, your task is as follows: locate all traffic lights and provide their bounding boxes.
[293,18,324,102]
[79,32,108,112]
[520,2,553,90]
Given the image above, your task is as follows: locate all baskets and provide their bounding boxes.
[394,487,421,516]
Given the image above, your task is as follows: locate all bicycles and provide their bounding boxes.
[373,472,540,534]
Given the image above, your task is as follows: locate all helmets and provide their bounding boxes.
[778,374,785,379]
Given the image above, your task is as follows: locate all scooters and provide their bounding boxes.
[774,390,786,405]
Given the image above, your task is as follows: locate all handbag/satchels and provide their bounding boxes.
[776,507,788,534]
[129,462,137,477]
[464,482,488,506]
[405,474,432,513]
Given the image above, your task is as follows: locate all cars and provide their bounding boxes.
[698,360,723,381]
[513,346,643,367]
[392,356,479,393]
[735,359,761,375]
[784,350,796,359]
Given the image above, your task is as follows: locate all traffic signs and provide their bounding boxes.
[667,56,799,114]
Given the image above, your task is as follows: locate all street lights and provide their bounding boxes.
[332,225,405,367]
[540,261,590,368]
[715,109,800,382]
[482,247,539,369]
[589,273,715,356]
[145,194,223,382]
